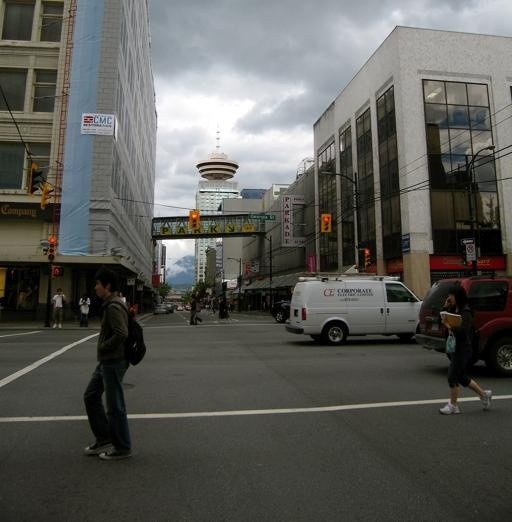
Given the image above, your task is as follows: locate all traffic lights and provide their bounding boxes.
[51,266,64,277]
[188,211,200,230]
[320,213,332,233]
[48,237,56,261]
[321,171,363,272]
[364,249,371,270]
[29,163,43,199]
[40,183,54,210]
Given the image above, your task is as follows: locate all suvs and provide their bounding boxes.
[415,272,512,377]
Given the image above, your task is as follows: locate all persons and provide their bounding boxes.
[189,293,202,325]
[117,291,128,303]
[213,294,231,324]
[431,283,492,417]
[52,287,67,328]
[83,262,136,461]
[78,292,91,328]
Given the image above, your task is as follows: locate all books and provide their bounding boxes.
[440,311,461,328]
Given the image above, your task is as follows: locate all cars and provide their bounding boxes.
[153,303,174,314]
[271,300,291,323]
[176,307,183,311]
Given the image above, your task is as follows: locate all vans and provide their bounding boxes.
[286,275,423,345]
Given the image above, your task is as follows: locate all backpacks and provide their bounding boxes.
[105,300,146,366]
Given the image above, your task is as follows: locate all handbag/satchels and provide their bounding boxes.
[446,336,456,353]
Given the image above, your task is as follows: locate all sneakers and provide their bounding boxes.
[84,442,134,460]
[440,402,460,415]
[481,390,492,409]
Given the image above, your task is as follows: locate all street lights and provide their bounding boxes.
[227,257,248,312]
[251,234,272,313]
[466,146,497,258]
